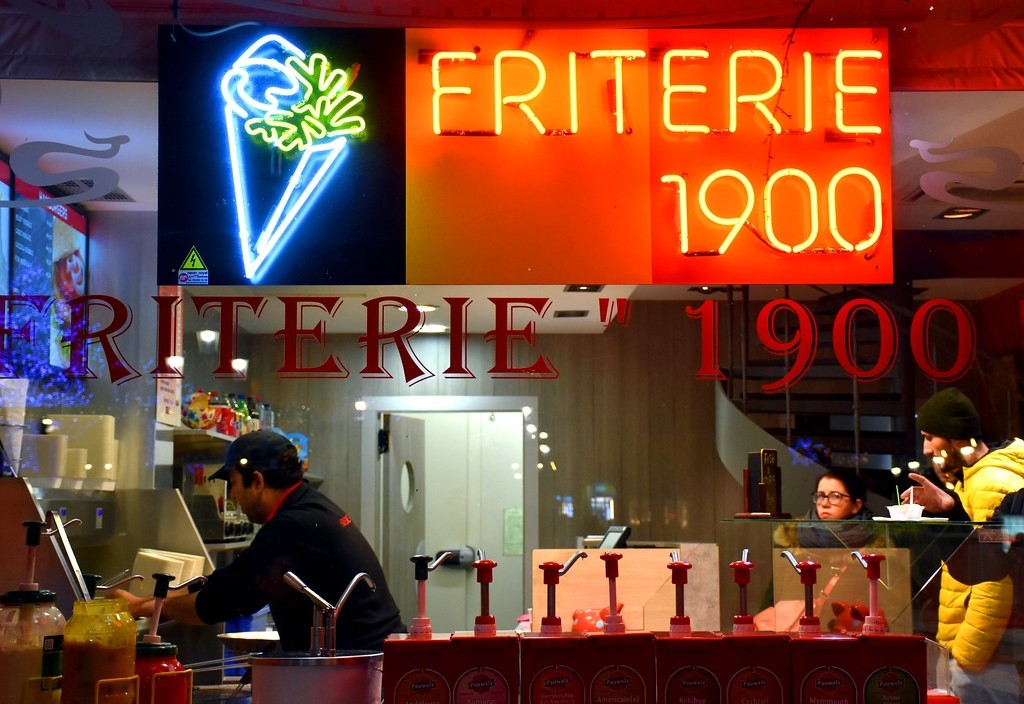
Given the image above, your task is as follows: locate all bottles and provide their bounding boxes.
[62,595,136,704]
[516,552,590,704]
[778,549,861,704]
[380,549,452,704]
[449,550,519,704]
[714,547,794,704]
[135,573,208,704]
[1,519,83,704]
[849,549,927,704]
[649,552,724,704]
[583,550,656,703]
[197,387,274,440]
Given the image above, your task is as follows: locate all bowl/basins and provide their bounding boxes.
[17,412,120,490]
[887,504,925,519]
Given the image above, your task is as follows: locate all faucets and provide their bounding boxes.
[324,572,376,657]
[95,574,145,591]
[167,575,209,591]
[742,548,751,562]
[557,550,588,575]
[669,551,680,563]
[780,550,801,575]
[40,518,82,536]
[850,550,867,569]
[476,548,487,562]
[427,551,456,572]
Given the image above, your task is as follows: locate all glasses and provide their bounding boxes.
[811,491,857,506]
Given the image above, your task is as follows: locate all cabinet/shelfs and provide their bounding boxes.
[171,397,325,704]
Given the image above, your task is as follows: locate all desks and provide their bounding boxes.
[719,509,1018,635]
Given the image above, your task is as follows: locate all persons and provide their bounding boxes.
[894,456,968,634]
[758,469,898,615]
[101,429,408,656]
[898,387,1023,704]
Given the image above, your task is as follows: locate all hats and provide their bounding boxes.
[208,430,298,481]
[916,387,982,441]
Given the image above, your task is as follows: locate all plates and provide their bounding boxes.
[871,514,949,522]
